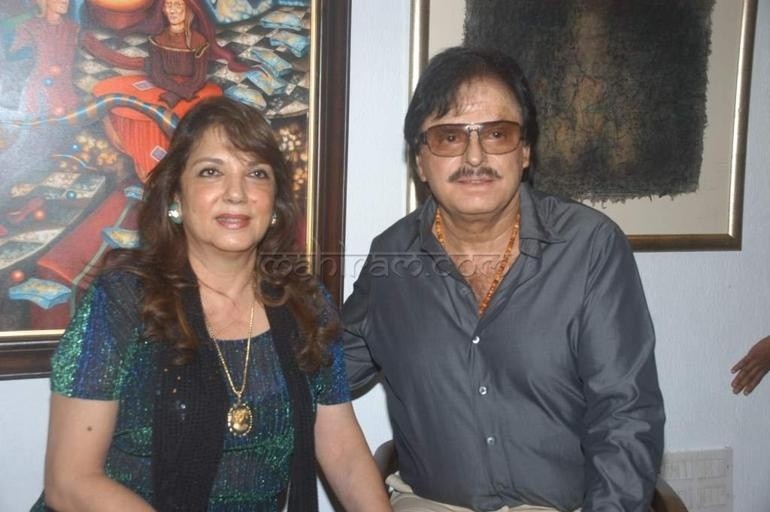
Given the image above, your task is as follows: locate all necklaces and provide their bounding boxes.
[205,300,258,439]
[434,206,521,317]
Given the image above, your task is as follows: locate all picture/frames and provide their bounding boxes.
[0,0,758,382]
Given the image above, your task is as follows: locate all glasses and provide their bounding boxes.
[414,120,527,157]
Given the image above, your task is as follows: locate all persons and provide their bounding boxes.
[339,46,665,511]
[0,0,152,230]
[141,0,249,108]
[30,93,396,512]
[729,334,770,396]
